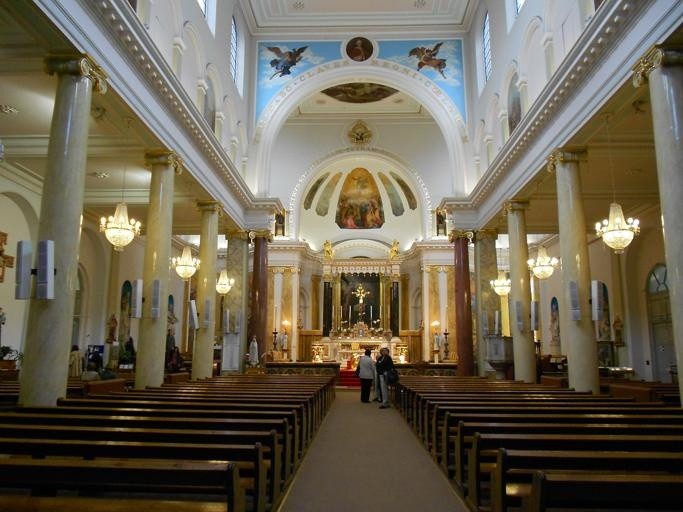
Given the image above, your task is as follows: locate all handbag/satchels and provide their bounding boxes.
[356,366,360,375]
[384,368,399,384]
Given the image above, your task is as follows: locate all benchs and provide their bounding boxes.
[0,372,341,512]
[387,375,683,512]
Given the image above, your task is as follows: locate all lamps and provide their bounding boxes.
[99,203,142,251]
[173,246,201,281]
[216,271,235,295]
[526,247,560,280]
[489,273,511,298]
[594,202,641,256]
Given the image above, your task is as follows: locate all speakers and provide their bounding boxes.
[515,301,524,331]
[234,310,241,333]
[15,239,31,299]
[151,279,160,319]
[223,310,229,334]
[130,278,143,318]
[203,300,211,328]
[188,300,199,329]
[569,280,582,321]
[591,279,603,320]
[36,240,55,300]
[531,301,540,330]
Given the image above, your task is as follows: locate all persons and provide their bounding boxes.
[409,41,446,81]
[68,345,83,378]
[263,45,306,79]
[124,337,137,373]
[358,347,393,409]
[80,362,102,395]
[166,329,174,366]
[340,197,382,229]
[323,240,334,256]
[167,346,184,371]
[389,241,401,257]
